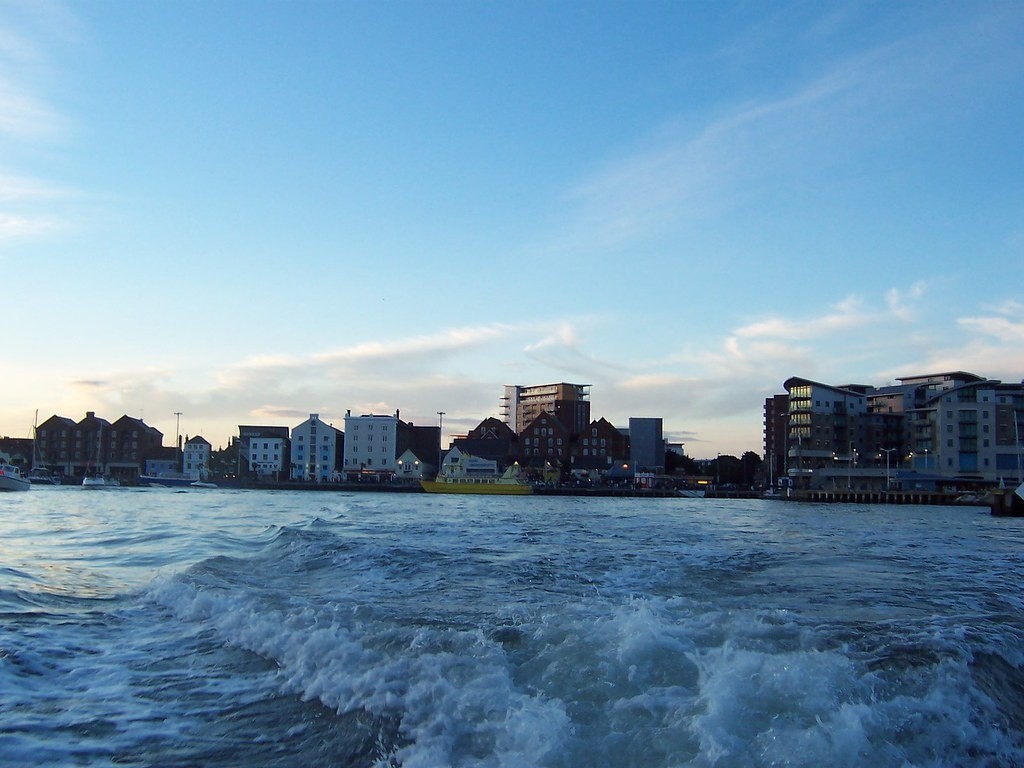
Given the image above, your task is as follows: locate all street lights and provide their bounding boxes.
[880,448,896,490]
[716,452,721,483]
[437,412,445,474]
[174,413,182,460]
[848,440,856,490]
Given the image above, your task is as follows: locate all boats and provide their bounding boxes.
[138,473,200,488]
[418,448,535,497]
[191,480,219,490]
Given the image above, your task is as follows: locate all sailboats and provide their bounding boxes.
[0,409,63,491]
[80,421,120,489]
